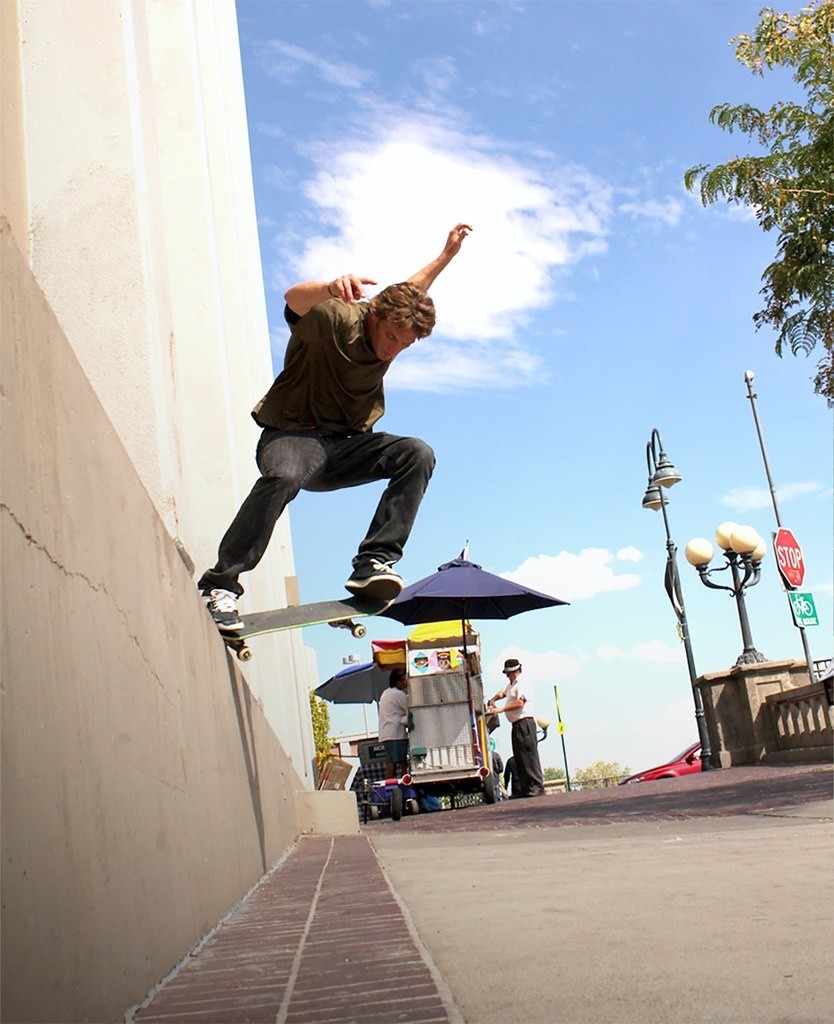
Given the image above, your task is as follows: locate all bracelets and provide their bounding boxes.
[328,281,340,298]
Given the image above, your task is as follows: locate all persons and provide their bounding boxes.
[376,667,410,778]
[487,659,544,796]
[504,756,523,798]
[196,220,472,630]
[490,740,504,786]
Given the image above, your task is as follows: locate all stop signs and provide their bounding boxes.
[773,527,805,588]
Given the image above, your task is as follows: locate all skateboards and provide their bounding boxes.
[219,594,398,662]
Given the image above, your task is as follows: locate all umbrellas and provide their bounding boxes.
[375,543,571,651]
[314,663,408,714]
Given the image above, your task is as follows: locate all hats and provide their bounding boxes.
[502,659,522,674]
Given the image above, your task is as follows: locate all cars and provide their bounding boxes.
[621,742,703,786]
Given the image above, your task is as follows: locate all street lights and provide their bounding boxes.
[743,371,817,683]
[642,427,715,775]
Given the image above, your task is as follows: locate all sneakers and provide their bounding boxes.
[343,558,405,600]
[202,587,244,630]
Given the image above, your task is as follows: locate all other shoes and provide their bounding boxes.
[525,790,543,796]
